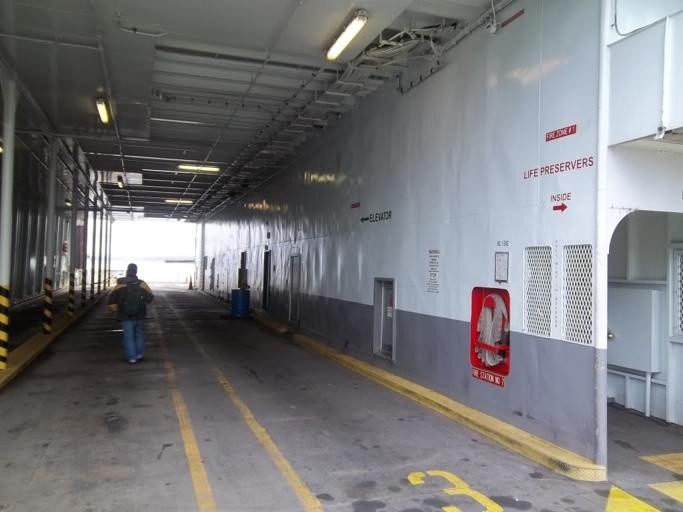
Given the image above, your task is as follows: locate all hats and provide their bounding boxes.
[127,264,137,274]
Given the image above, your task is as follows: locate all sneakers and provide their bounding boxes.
[128,356,143,364]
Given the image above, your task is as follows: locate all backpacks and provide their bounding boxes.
[123,286,143,317]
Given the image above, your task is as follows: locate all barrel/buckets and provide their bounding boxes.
[232,289,250,318]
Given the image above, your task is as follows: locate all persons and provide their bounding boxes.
[108,263,154,364]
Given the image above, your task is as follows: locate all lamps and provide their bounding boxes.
[95,97,110,126]
[325,9,369,62]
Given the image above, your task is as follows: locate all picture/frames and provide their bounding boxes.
[495,252,509,282]
